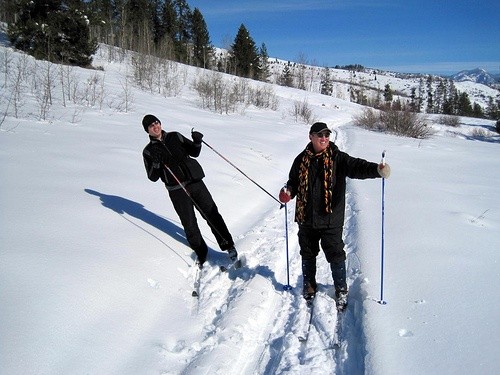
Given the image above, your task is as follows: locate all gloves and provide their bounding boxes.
[192,131,204,143]
[150,146,163,160]
[279,187,291,203]
[377,163,390,178]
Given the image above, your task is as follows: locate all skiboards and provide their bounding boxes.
[297,298,342,349]
[193,260,237,297]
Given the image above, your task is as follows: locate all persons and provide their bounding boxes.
[141,113,237,262]
[279,122,391,310]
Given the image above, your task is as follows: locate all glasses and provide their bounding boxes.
[317,132,330,137]
[150,121,158,128]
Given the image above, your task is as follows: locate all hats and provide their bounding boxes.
[142,115,161,133]
[309,122,332,134]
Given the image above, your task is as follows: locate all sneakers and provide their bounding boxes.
[303,282,316,300]
[228,246,237,261]
[335,291,348,311]
